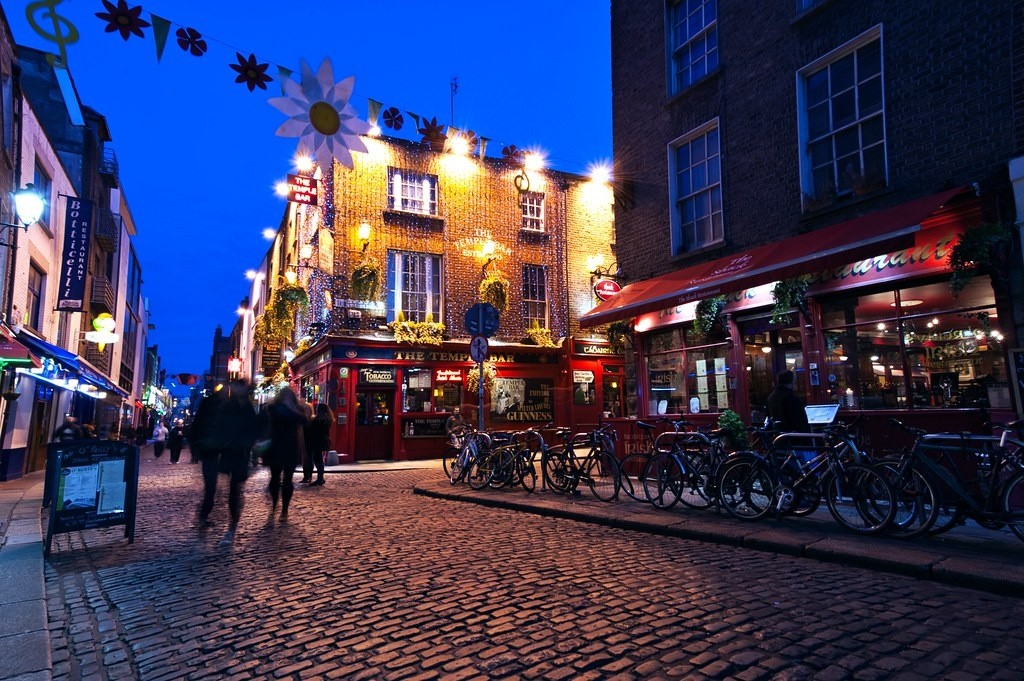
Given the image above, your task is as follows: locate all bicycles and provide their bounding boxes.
[441,410,1024,545]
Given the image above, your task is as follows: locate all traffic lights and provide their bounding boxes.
[160,371,165,384]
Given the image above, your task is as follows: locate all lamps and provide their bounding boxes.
[359,223,370,252]
[0,183,43,250]
[482,241,497,273]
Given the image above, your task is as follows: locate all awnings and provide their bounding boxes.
[578,182,982,330]
[0,319,129,400]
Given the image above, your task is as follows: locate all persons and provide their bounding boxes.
[446,407,472,449]
[51,417,188,463]
[574,383,590,406]
[766,369,823,503]
[188,381,335,522]
[494,381,521,415]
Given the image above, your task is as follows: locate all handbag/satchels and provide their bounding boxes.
[252,435,272,454]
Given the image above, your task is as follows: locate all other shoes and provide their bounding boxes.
[298,476,313,483]
[308,478,326,487]
